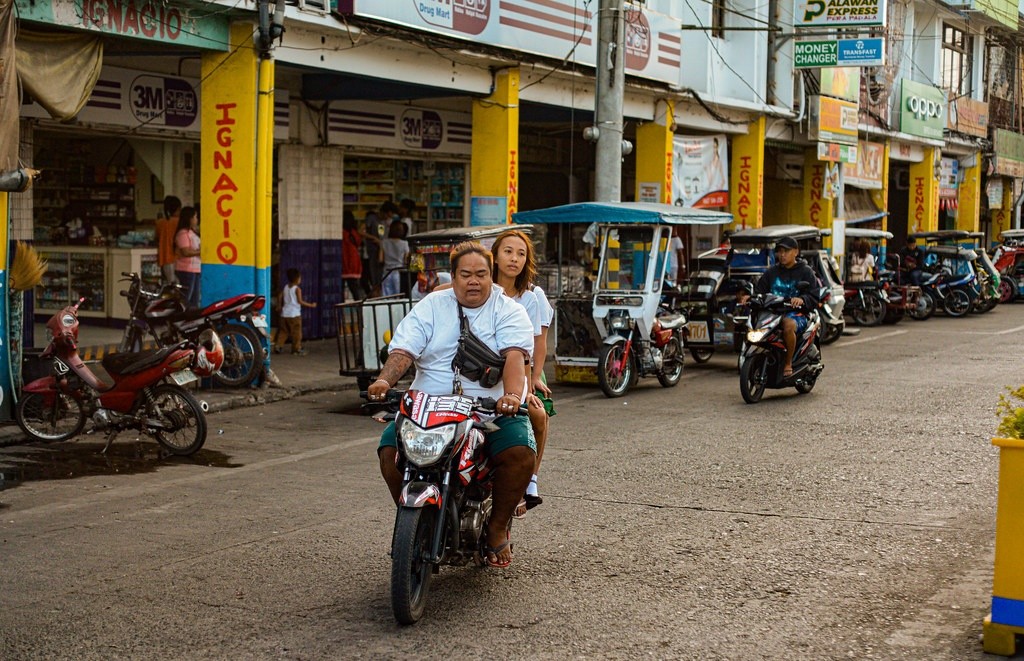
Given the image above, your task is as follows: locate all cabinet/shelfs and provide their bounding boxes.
[29,160,161,317]
[344,151,467,269]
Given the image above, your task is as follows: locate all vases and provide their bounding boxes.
[983,436,1024,653]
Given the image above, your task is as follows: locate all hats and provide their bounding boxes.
[907,235,916,243]
[776,236,798,250]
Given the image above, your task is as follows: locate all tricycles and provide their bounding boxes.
[817,225,1024,326]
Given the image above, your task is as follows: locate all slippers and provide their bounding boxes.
[512,500,528,518]
[486,530,511,567]
[784,367,793,376]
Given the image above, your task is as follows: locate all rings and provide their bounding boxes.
[370,394,375,399]
[501,403,509,409]
[509,405,515,410]
[375,395,380,399]
[381,393,386,398]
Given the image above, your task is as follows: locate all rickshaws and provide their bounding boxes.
[510,198,734,398]
[685,225,846,365]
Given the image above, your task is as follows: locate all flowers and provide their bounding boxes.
[993,384,1024,441]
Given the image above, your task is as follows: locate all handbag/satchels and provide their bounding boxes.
[452,316,505,388]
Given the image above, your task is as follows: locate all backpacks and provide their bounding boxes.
[850,252,869,282]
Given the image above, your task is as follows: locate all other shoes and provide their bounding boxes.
[523,480,542,509]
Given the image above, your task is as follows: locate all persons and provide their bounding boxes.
[366,240,537,569]
[274,268,317,357]
[667,224,687,309]
[433,230,556,520]
[173,206,200,309]
[153,195,181,283]
[705,137,725,191]
[740,235,821,378]
[359,198,416,299]
[342,210,364,301]
[851,241,875,282]
[376,220,409,298]
[714,229,736,254]
[895,235,923,286]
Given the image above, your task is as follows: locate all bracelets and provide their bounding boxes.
[374,379,391,387]
[504,392,520,400]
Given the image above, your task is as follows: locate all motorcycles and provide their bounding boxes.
[14,296,208,458]
[358,384,544,627]
[736,280,825,405]
[115,271,266,390]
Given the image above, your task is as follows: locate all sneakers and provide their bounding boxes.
[292,348,310,356]
[273,346,282,355]
